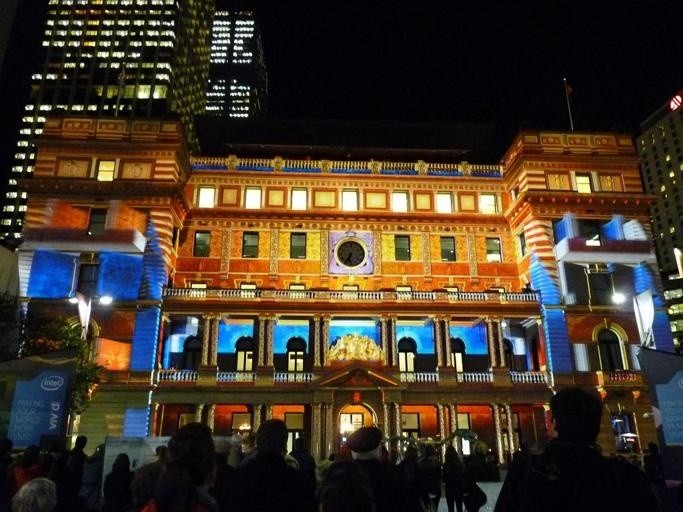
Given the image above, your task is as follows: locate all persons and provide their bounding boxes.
[1,389,683,511]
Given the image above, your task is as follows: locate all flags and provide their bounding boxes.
[565,82,573,96]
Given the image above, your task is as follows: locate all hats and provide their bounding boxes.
[347,427,383,453]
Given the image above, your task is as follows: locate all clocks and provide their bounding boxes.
[333,236,367,269]
